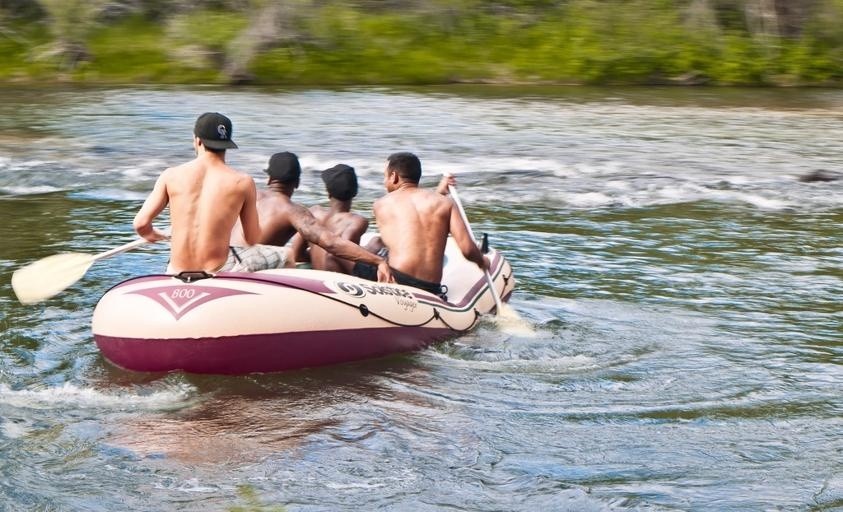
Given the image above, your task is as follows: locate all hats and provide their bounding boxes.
[263,151,300,180]
[194,113,238,149]
[321,164,357,200]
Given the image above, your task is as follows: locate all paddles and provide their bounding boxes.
[11,228,172,306]
[442,171,534,336]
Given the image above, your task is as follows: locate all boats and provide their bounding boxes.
[91,238,515,373]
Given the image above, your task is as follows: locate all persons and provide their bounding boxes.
[371,152,491,296]
[285,163,385,277]
[133,111,296,277]
[229,152,398,285]
